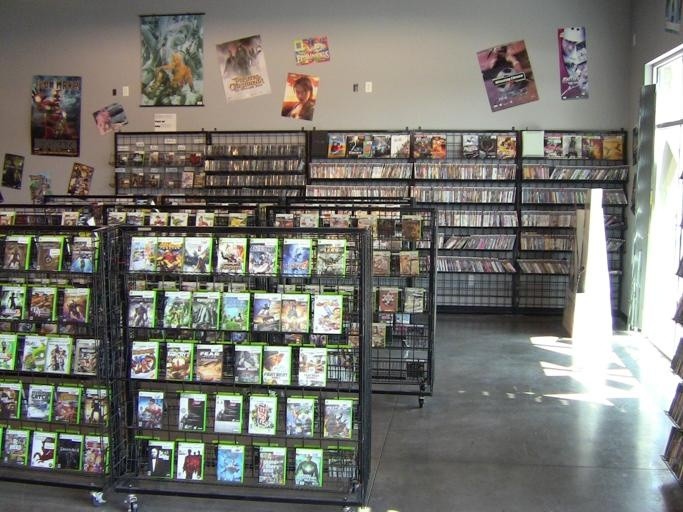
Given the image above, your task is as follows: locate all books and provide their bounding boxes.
[663,255,682,487]
[436,130,628,272]
[0,232,110,474]
[116,147,205,204]
[108,211,248,228]
[1,211,80,227]
[275,213,351,229]
[357,214,426,348]
[204,143,305,207]
[304,160,436,203]
[124,233,353,489]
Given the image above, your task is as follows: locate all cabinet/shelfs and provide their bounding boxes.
[0,193,440,510]
[113,128,628,317]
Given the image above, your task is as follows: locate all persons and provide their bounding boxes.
[282,76,313,122]
